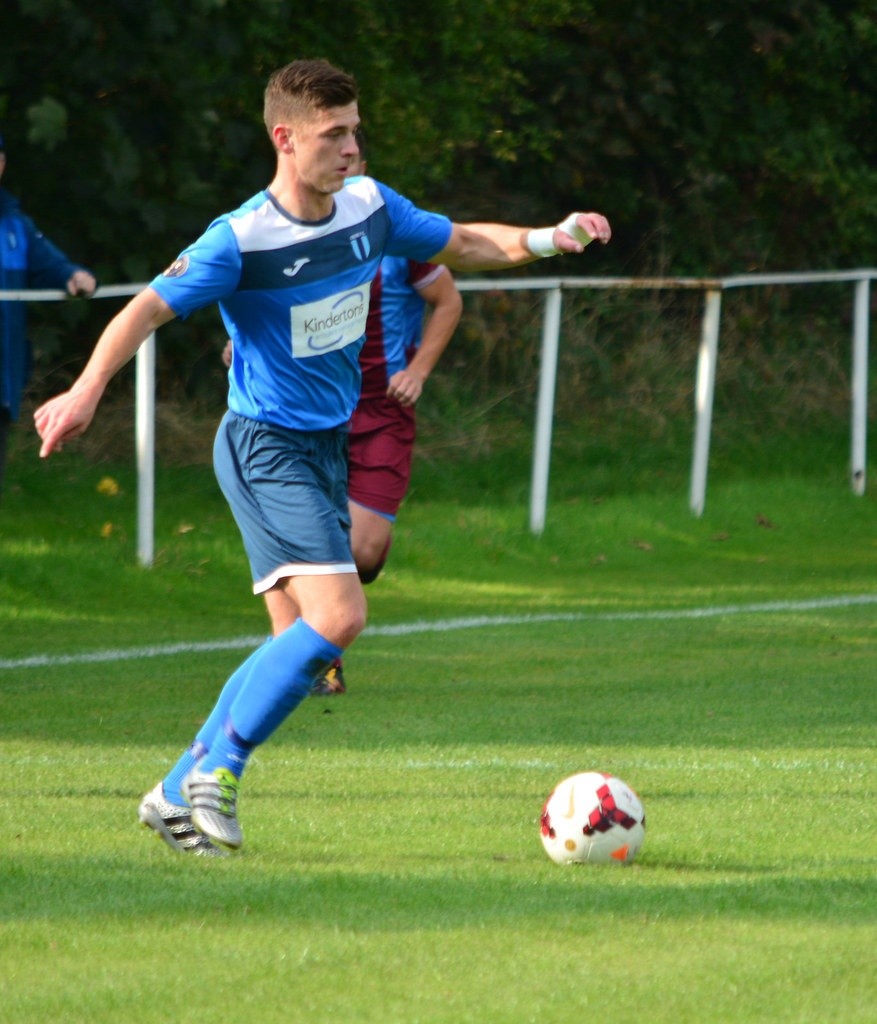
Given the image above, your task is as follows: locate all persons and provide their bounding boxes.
[225,130,462,695]
[0,131,97,487]
[33,58,611,863]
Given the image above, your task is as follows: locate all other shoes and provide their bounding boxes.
[313,662,346,693]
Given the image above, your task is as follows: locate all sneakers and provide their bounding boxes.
[180,761,243,850]
[137,781,220,857]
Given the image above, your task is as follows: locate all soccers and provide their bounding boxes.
[538,770,647,868]
